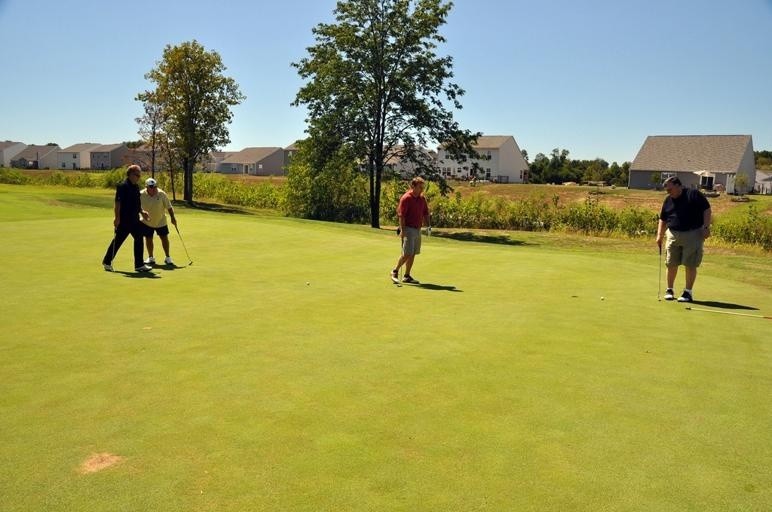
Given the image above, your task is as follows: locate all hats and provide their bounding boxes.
[145,178,156,186]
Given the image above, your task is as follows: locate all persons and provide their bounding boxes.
[656,176,712,302]
[101,165,153,272]
[139,178,177,267]
[391,177,431,284]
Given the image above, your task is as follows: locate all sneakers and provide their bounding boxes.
[402,276,419,283]
[135,257,155,271]
[165,256,172,265]
[678,291,691,301]
[391,270,400,283]
[665,289,673,299]
[102,262,113,271]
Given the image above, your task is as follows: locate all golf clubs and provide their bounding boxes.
[397,237,402,287]
[175,224,193,265]
[113,225,117,272]
[685,308,772,319]
[657,246,661,301]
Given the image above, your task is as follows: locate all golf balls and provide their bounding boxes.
[601,297,604,299]
[307,282,310,285]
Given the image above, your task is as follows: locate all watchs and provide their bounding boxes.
[703,224,710,232]
